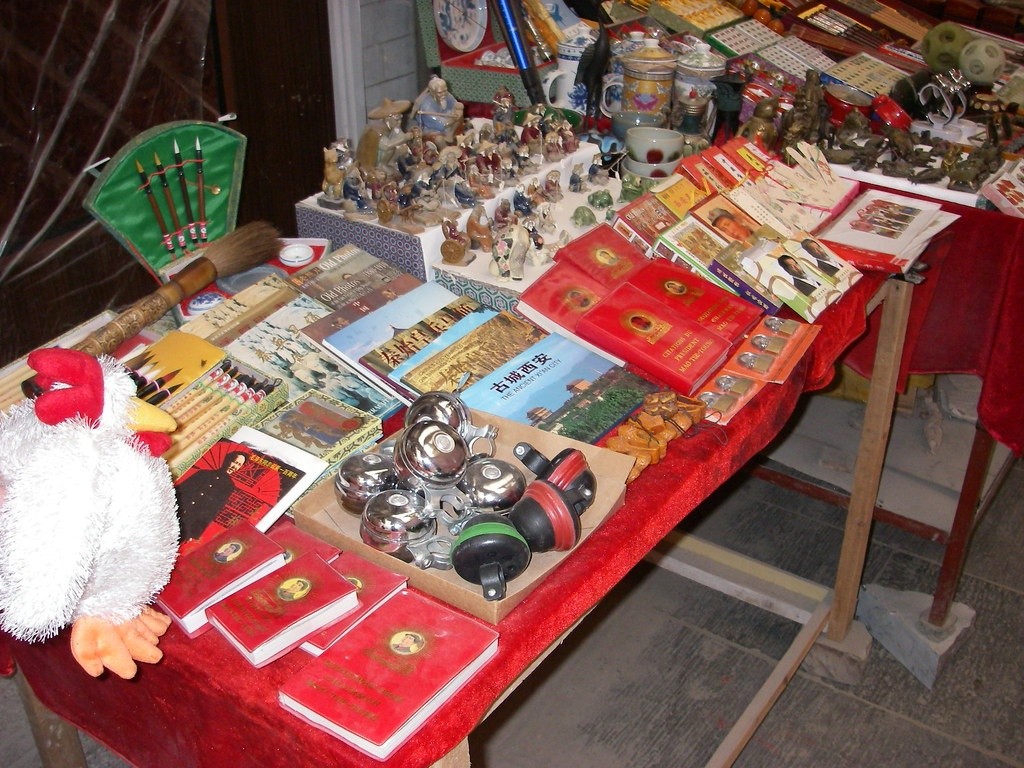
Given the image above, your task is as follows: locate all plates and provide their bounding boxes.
[433,1,488,53]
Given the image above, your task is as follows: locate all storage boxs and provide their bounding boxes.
[414,0,746,107]
[286,404,634,625]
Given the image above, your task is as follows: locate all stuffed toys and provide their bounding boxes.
[0,349,181,679]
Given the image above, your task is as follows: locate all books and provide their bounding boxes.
[143,136,965,761]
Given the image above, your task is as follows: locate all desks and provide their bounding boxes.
[0,101,1022,768]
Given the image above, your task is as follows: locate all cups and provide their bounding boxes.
[541,25,726,139]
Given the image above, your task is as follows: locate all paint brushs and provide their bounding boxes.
[153,152,188,250]
[135,159,175,253]
[174,138,198,243]
[21,218,286,398]
[195,136,208,243]
[114,345,283,469]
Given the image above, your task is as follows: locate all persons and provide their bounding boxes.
[778,255,821,297]
[346,77,609,257]
[707,208,755,243]
[174,451,248,546]
[800,239,844,277]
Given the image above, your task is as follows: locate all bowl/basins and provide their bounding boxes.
[823,82,873,128]
[611,112,664,138]
[621,127,684,202]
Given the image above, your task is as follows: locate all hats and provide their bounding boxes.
[708,207,734,226]
